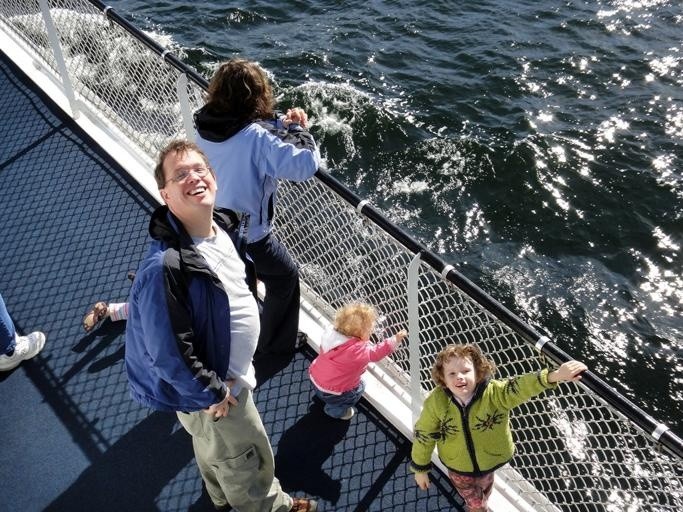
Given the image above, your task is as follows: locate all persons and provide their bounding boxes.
[81,301,128,332]
[407,341,587,512]
[307,300,408,420]
[124,137,319,512]
[193,55,319,355]
[0,293,45,376]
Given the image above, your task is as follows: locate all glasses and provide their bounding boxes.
[160,164,212,191]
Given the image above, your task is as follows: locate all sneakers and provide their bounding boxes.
[291,494,317,512]
[215,500,231,512]
[83,301,108,334]
[258,332,308,354]
[0,331,46,372]
[323,404,354,421]
